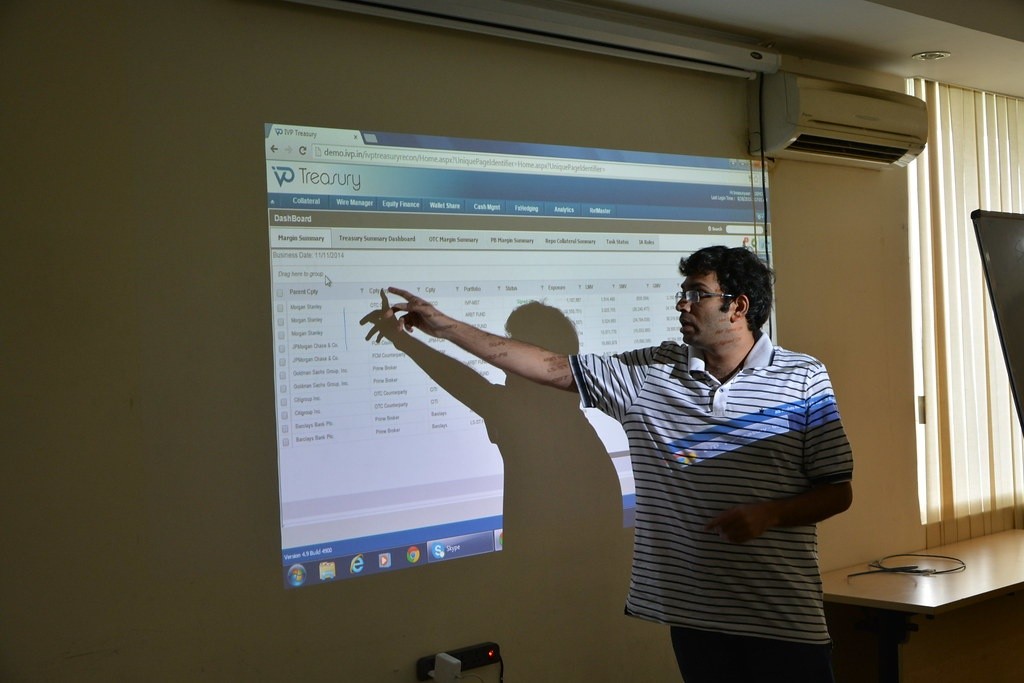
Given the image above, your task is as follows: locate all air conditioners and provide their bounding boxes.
[748,72,929,171]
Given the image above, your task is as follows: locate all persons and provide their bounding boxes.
[387,246,855,683]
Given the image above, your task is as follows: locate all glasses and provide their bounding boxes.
[675,291,738,303]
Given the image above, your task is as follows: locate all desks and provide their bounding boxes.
[814,527,1024,682]
